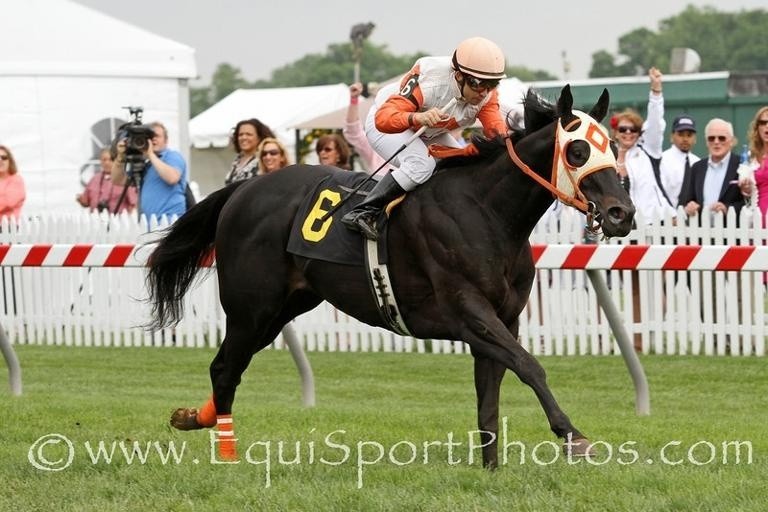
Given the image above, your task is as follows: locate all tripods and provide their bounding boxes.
[63,154,147,330]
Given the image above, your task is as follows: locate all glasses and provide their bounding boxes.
[708,136,726,142]
[466,75,500,94]
[617,126,638,132]
[0,156,7,160]
[261,150,282,156]
[758,120,768,125]
[318,147,335,153]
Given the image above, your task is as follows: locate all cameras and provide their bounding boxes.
[97,200,110,213]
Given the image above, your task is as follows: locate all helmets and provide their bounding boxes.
[451,37,508,80]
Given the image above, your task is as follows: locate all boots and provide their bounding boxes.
[341,169,406,241]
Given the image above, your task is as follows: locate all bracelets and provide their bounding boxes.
[649,87,662,93]
[350,97,359,105]
[114,158,125,169]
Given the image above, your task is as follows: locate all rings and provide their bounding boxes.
[744,185,750,189]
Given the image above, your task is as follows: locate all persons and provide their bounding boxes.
[342,36,513,242]
[676,117,743,246]
[223,118,278,187]
[75,143,138,216]
[658,115,702,208]
[109,121,187,233]
[736,108,768,285]
[316,133,353,172]
[450,127,473,148]
[0,144,27,247]
[608,67,667,355]
[255,137,290,175]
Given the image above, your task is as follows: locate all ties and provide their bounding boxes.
[685,156,690,175]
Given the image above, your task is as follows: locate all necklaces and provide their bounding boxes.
[616,160,626,169]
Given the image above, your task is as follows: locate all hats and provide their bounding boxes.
[672,116,697,132]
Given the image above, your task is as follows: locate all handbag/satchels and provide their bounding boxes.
[185,182,196,211]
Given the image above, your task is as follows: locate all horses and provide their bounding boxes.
[136,83,638,472]
